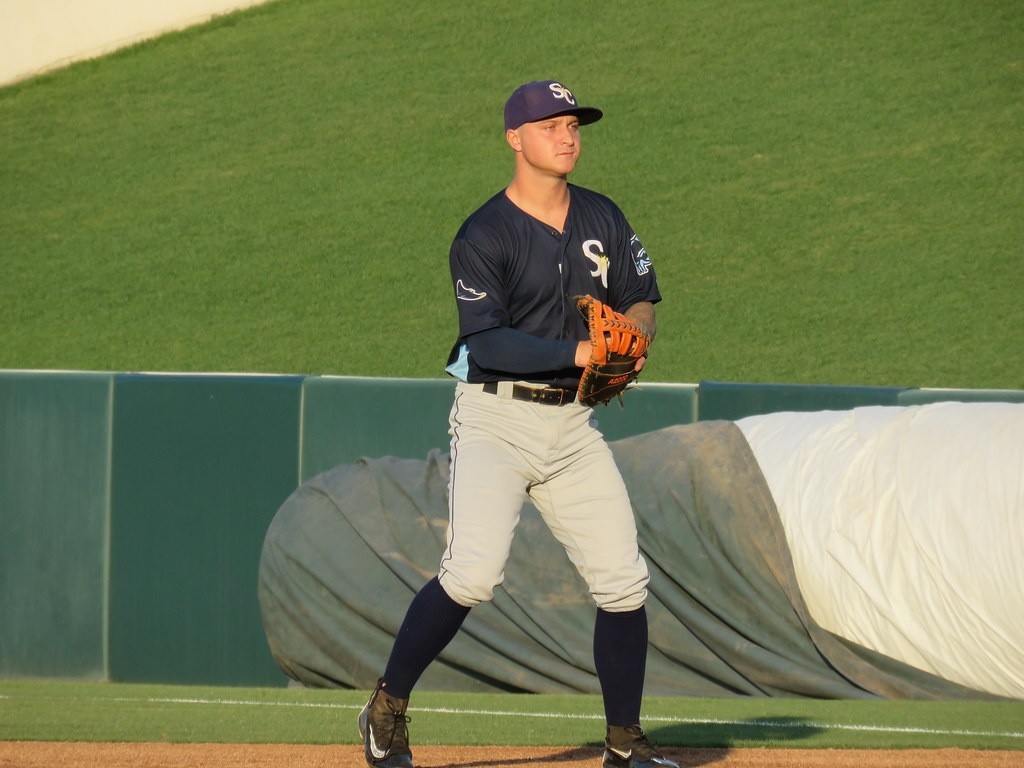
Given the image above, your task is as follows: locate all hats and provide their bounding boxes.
[503,81,603,131]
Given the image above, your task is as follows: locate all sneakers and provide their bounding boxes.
[357,677,415,768]
[603,724,679,768]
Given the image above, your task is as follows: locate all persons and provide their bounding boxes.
[359,76,682,768]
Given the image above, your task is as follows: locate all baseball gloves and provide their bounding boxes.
[575,293,653,409]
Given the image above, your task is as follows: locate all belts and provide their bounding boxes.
[484,381,578,407]
